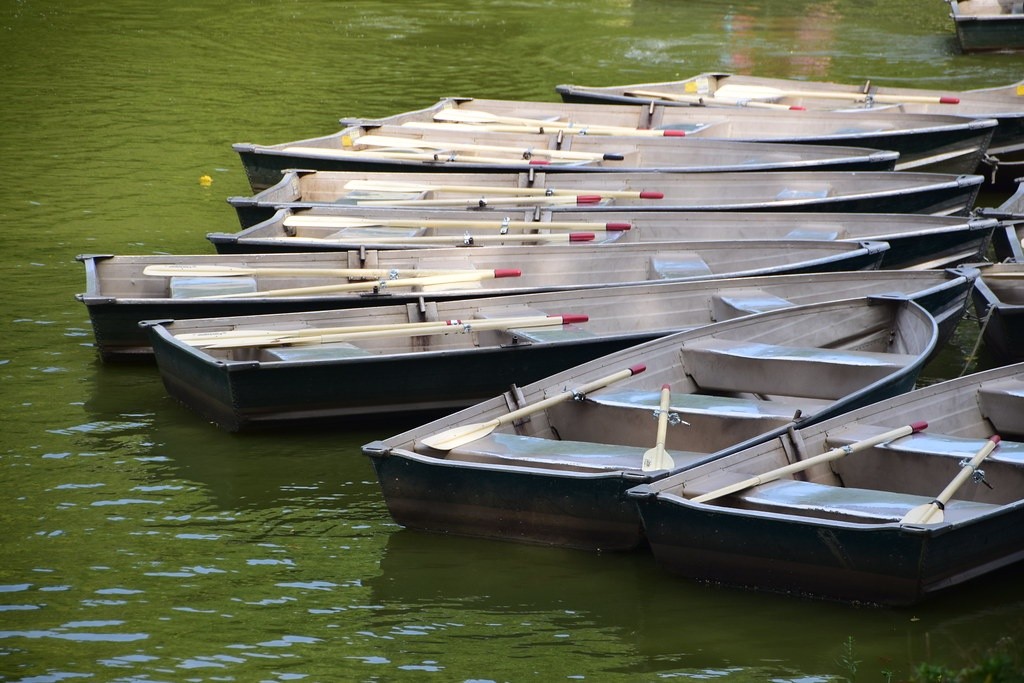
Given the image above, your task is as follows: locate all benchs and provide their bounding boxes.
[546,143,643,168]
[645,249,714,281]
[977,379,1024,435]
[324,225,428,239]
[544,378,836,454]
[414,431,712,474]
[825,422,1024,505]
[516,114,570,123]
[707,287,800,322]
[683,468,1002,525]
[652,114,730,138]
[832,101,928,115]
[335,182,430,201]
[544,223,637,244]
[780,223,846,240]
[474,303,598,347]
[549,196,619,206]
[356,146,462,157]
[776,183,833,201]
[680,336,918,400]
[228,320,374,362]
[738,154,774,165]
[414,257,483,291]
[165,274,258,298]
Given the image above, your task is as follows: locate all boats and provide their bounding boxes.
[75,64,1023,437]
[942,0,1024,56]
[356,289,941,555]
[625,361,1024,606]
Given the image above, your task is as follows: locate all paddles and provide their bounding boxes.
[714,84,960,105]
[433,108,650,130]
[899,435,1002,524]
[142,264,506,278]
[201,314,589,349]
[174,312,570,348]
[283,216,631,230]
[624,90,806,110]
[344,180,664,200]
[641,384,674,472]
[689,420,928,503]
[282,146,552,165]
[357,195,602,206]
[187,269,523,299]
[262,233,596,244]
[402,122,686,137]
[421,363,647,450]
[353,135,625,161]
